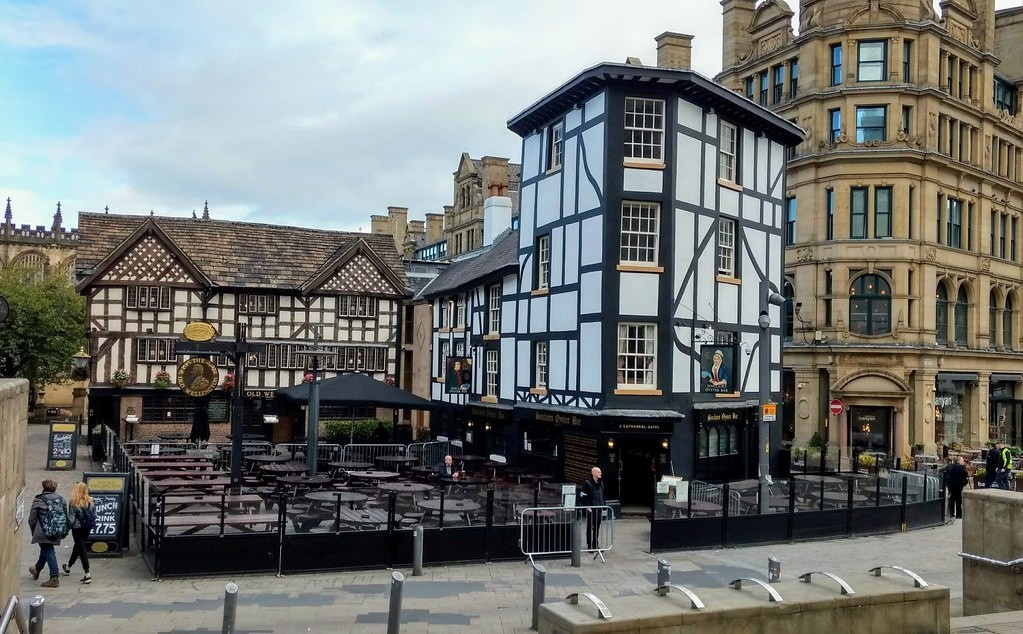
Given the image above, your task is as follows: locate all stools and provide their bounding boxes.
[243,483,495,533]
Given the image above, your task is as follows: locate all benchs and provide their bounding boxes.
[167,526,276,535]
[164,508,255,514]
[172,489,224,491]
[163,491,227,495]
[139,468,186,472]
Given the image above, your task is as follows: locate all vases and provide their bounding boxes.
[117,381,129,386]
[155,379,169,388]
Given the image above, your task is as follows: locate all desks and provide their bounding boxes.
[138,447,185,452]
[795,474,843,498]
[229,439,272,445]
[157,433,191,443]
[131,456,582,522]
[810,492,869,502]
[662,500,722,512]
[865,486,920,501]
[222,446,266,453]
[739,496,800,508]
[226,435,264,438]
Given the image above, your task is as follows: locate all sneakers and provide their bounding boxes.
[62,564,70,575]
[80,575,92,584]
[29,566,39,580]
[41,576,59,587]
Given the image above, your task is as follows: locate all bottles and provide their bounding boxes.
[460,471,467,480]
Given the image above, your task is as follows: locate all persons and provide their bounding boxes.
[945,456,968,518]
[439,455,458,489]
[582,467,604,553]
[985,443,1003,488]
[28,479,67,587]
[995,442,1012,491]
[368,420,391,445]
[62,481,98,584]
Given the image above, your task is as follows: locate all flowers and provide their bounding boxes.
[384,376,394,384]
[111,369,131,381]
[155,371,170,380]
[301,374,312,383]
[220,374,235,389]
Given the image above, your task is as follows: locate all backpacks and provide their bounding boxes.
[75,503,99,530]
[37,495,68,539]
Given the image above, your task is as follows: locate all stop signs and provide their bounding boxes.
[831,399,842,414]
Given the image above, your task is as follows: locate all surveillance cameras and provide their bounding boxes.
[798,385,802,390]
[745,349,751,355]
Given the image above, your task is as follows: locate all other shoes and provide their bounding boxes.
[593,546,604,552]
[587,547,594,553]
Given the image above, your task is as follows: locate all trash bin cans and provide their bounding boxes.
[102,462,113,472]
[35,403,47,424]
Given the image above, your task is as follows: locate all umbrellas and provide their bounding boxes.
[189,393,211,449]
[272,373,440,452]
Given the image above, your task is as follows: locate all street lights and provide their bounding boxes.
[293,343,340,471]
[69,345,91,425]
[758,280,786,514]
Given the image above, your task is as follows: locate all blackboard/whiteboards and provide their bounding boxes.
[49,432,75,460]
[88,490,123,540]
